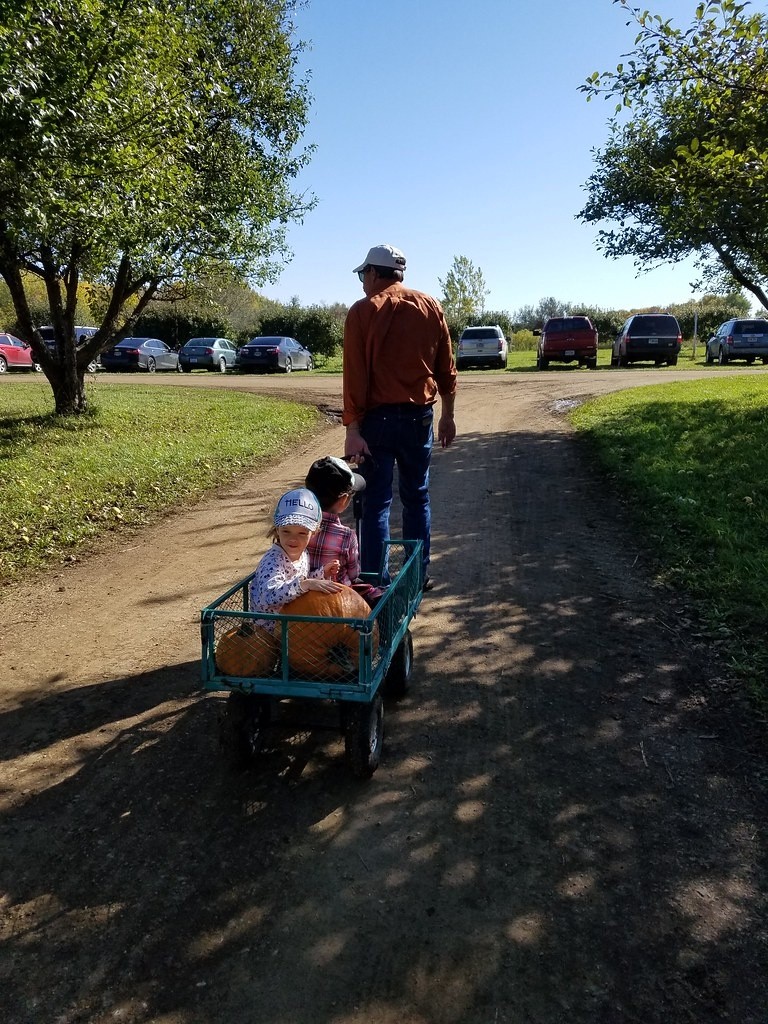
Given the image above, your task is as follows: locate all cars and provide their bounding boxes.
[456,326,511,371]
[178,338,239,373]
[240,336,313,374]
[100,337,184,373]
[0,333,44,374]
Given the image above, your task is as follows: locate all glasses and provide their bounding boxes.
[358,269,365,282]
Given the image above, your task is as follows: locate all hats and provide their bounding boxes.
[353,244,407,273]
[305,456,366,493]
[274,485,322,532]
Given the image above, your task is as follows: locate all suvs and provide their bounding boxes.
[610,313,683,367]
[533,315,598,369]
[705,317,768,366]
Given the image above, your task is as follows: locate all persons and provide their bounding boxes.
[250,488,342,632]
[305,456,405,624]
[343,243,457,591]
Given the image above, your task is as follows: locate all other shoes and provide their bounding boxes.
[422,579,435,592]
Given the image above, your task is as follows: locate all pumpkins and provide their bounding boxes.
[215,621,276,677]
[274,582,379,676]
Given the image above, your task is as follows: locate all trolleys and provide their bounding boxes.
[196,453,427,779]
